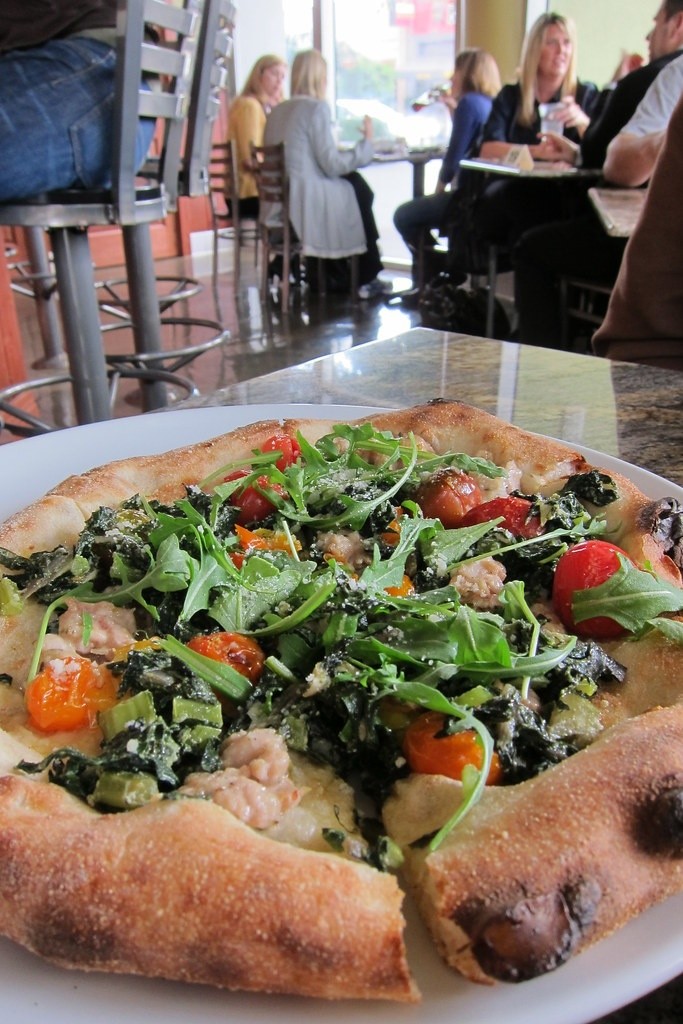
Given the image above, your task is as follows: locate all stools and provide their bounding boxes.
[0,0,237,435]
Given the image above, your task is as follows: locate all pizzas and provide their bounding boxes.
[0,394,683,1000]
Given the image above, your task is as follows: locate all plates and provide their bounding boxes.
[1,401,683,1024]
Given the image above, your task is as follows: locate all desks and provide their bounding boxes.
[345,144,450,304]
[589,187,649,237]
[141,328,683,1024]
[456,157,603,338]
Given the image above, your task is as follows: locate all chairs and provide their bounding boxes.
[248,141,354,314]
[202,141,263,308]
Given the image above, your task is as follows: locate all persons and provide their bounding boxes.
[471,0,683,370]
[0,0,158,199]
[260,50,392,306]
[222,54,298,284]
[394,47,502,305]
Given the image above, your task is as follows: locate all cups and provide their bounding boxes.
[538,102,564,142]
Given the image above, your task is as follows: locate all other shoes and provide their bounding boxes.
[401,287,424,302]
[365,280,393,298]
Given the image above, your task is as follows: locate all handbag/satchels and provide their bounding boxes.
[422,285,511,342]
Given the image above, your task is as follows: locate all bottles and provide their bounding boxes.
[412,83,451,112]
